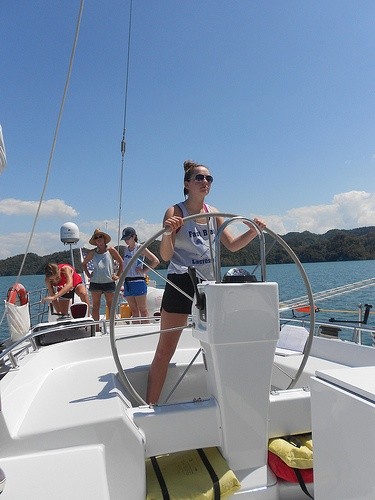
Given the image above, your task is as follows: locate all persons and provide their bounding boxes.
[121,227,160,324]
[143,161,266,406]
[83,228,124,331]
[41,264,91,318]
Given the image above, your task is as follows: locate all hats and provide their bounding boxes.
[89,228,111,245]
[119,227,135,240]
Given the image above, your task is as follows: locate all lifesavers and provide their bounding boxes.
[7,282,28,305]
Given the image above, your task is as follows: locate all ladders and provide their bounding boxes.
[279,277,375,311]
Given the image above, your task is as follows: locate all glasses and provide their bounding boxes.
[94,235,103,239]
[49,276,60,281]
[188,174,213,183]
